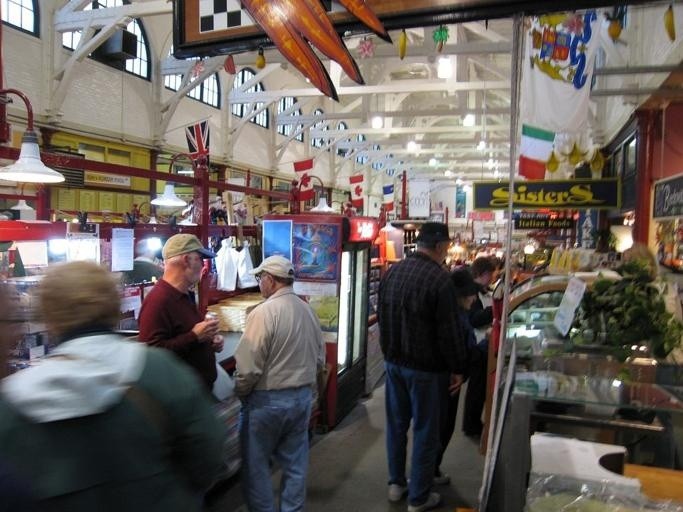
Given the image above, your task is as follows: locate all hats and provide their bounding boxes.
[451,269,483,297]
[248,256,296,279]
[161,233,216,260]
[39,260,120,336]
[412,223,451,242]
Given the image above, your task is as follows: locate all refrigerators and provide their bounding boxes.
[260,214,380,436]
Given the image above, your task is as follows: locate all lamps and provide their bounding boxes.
[292,175,335,214]
[0,80,65,187]
[148,151,198,210]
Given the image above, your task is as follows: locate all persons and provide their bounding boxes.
[1,285,28,380]
[432,252,504,487]
[137,233,223,394]
[1,259,229,511]
[622,243,658,283]
[128,240,166,282]
[376,223,463,511]
[233,255,326,512]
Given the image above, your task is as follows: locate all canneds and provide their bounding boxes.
[204,312,217,322]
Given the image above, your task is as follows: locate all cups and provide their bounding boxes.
[77,210,87,224]
[102,209,111,223]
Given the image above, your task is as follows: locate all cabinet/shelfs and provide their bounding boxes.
[444,214,474,272]
[370,215,420,322]
[0,217,370,446]
[481,226,683,512]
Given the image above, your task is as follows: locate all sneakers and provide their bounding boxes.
[388,478,411,502]
[407,492,441,511]
[432,465,451,484]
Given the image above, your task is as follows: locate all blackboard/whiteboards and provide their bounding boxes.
[649,173,683,221]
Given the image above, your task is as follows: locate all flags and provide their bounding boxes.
[293,159,316,203]
[186,120,209,173]
[519,15,600,181]
[349,174,365,209]
[382,183,394,212]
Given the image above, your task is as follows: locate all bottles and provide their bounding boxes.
[130,203,137,222]
